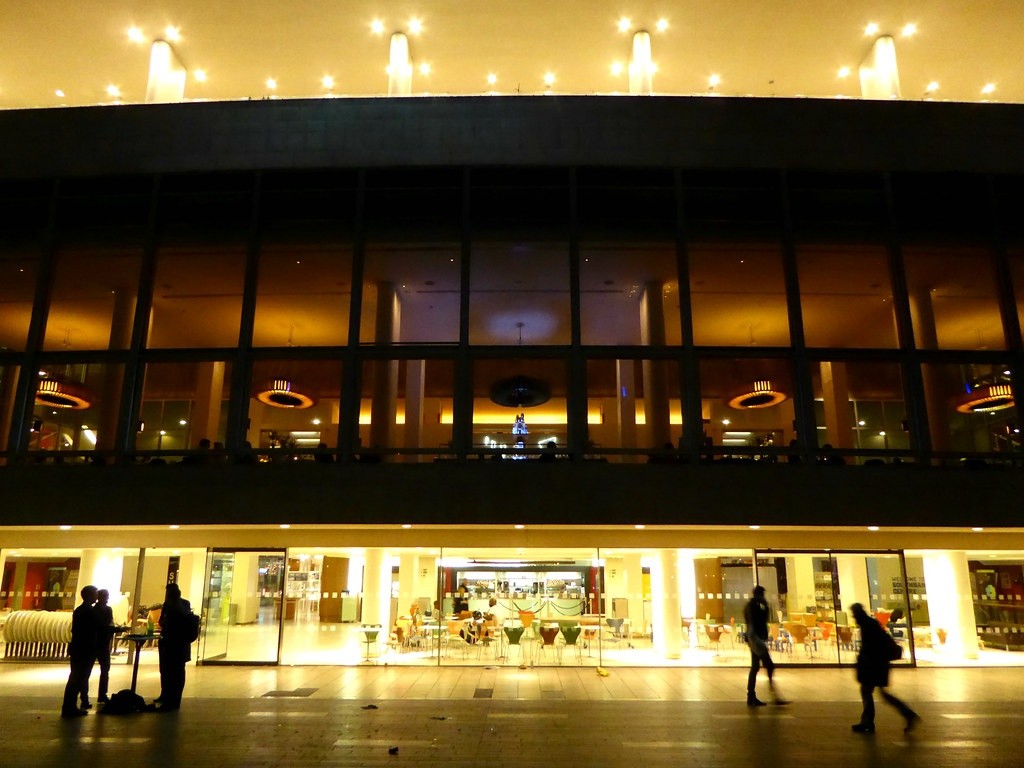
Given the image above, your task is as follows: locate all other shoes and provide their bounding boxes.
[852,723,875,736]
[96,696,111,703]
[81,703,93,710]
[775,699,791,705]
[153,696,161,704]
[69,708,88,718]
[904,713,920,733]
[747,698,768,707]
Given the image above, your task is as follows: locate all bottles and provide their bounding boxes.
[134,622,153,636]
[985,585,996,598]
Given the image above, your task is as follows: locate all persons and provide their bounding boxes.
[454,585,468,613]
[850,602,921,733]
[79,590,115,708]
[787,439,801,463]
[61,586,98,718]
[233,440,259,461]
[314,443,334,462]
[538,441,557,459]
[822,444,848,463]
[182,439,227,462]
[152,582,190,712]
[43,571,60,611]
[743,585,792,706]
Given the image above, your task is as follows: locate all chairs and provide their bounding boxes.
[681,610,893,653]
[361,611,624,666]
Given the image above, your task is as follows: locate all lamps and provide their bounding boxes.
[488,322,552,407]
[937,327,1015,414]
[254,321,320,409]
[35,326,97,410]
[719,326,792,410]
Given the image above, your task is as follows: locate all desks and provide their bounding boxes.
[486,626,506,658]
[419,625,448,660]
[97,635,162,712]
[807,626,827,651]
[574,625,602,659]
[352,626,383,666]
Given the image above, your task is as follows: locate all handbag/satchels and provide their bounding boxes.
[190,611,200,643]
[886,632,902,661]
